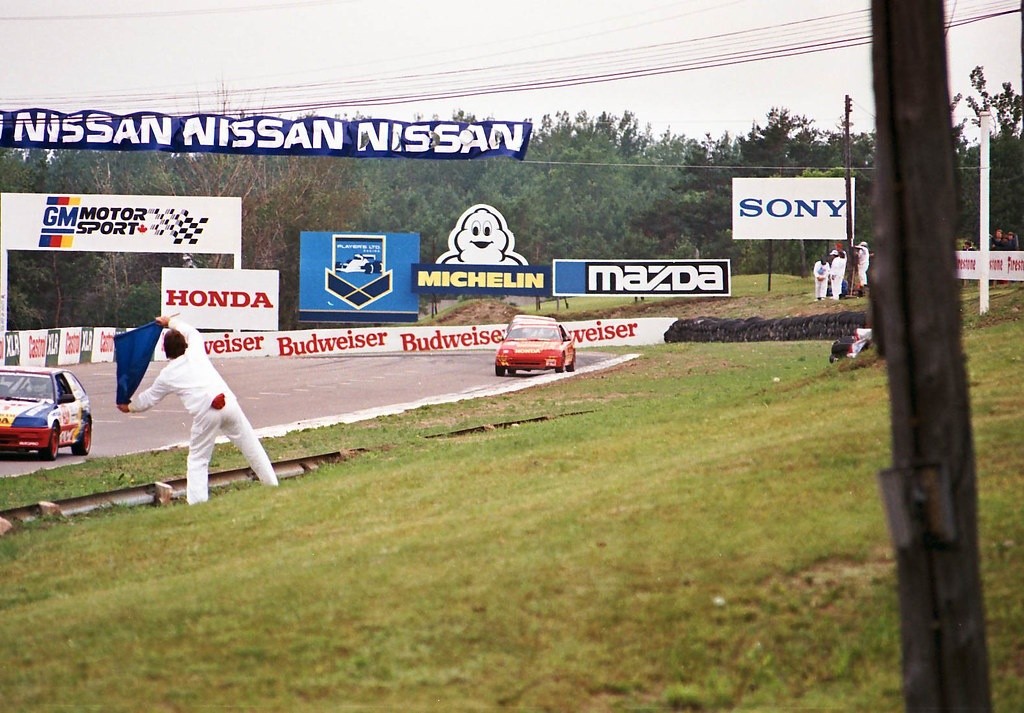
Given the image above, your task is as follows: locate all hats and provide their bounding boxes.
[860,242,867,246]
[830,250,838,255]
[836,244,843,247]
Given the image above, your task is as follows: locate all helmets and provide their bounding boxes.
[31,377,48,392]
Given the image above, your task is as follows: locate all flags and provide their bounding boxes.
[112,320,164,405]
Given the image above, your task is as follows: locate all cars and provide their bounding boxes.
[0,366,92,460]
[494,315,575,376]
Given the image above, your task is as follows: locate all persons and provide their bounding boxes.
[962,230,1016,284]
[117,316,278,505]
[29,378,50,397]
[813,241,869,301]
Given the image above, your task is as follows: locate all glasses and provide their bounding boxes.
[31,381,46,385]
